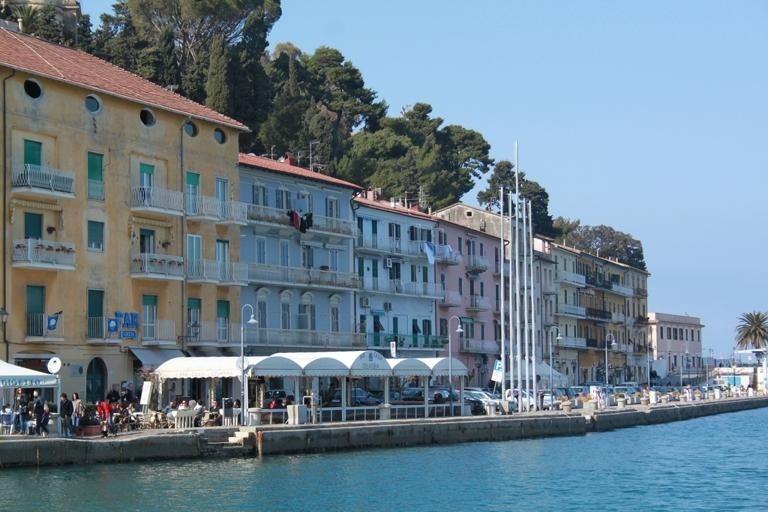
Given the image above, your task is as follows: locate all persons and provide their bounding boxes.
[59,392,73,437]
[95,380,142,438]
[40,399,50,437]
[71,392,86,437]
[281,398,292,408]
[0,403,19,434]
[269,399,278,408]
[162,396,241,427]
[29,389,50,437]
[10,387,28,435]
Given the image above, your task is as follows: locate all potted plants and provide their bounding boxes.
[82,417,101,435]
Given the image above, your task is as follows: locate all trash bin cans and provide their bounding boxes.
[563,403,572,413]
[617,398,625,408]
[591,401,598,410]
[248,407,260,426]
[488,404,497,418]
[695,394,700,402]
[222,397,234,425]
[722,393,727,400]
[679,396,686,404]
[709,393,715,401]
[662,396,669,405]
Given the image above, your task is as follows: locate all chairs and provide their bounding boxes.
[1,414,11,433]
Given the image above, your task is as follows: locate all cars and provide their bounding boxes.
[378,387,731,415]
[264,389,294,409]
[331,388,380,407]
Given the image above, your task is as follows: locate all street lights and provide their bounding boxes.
[550,326,563,411]
[240,303,258,425]
[605,334,617,384]
[449,316,464,383]
[680,343,689,385]
